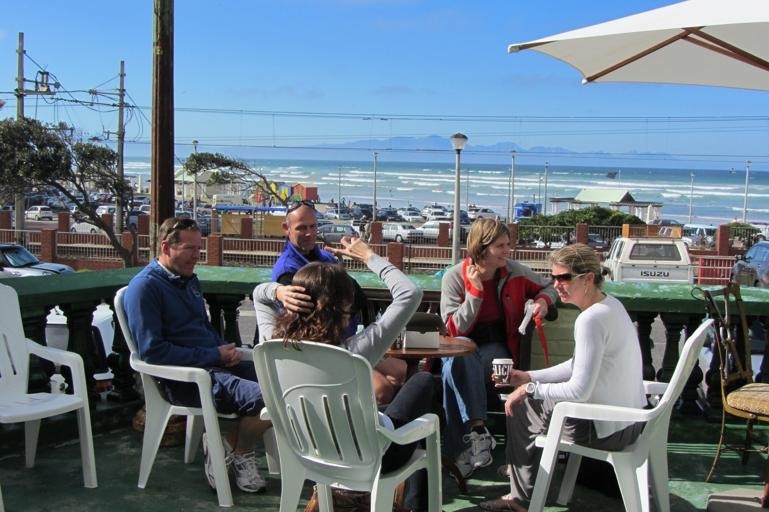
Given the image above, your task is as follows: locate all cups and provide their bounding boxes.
[492,358,514,387]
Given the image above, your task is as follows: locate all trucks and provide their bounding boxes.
[211,194,252,215]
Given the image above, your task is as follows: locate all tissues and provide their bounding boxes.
[403,306,443,349]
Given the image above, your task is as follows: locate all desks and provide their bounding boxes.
[2,251,768,446]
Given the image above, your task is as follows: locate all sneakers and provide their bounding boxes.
[497,465,511,481]
[203,432,235,488]
[480,493,530,512]
[454,447,493,477]
[462,425,497,467]
[230,451,267,492]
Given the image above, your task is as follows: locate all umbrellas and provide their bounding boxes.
[507,1,768,94]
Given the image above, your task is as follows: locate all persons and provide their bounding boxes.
[438,215,561,484]
[475,242,650,510]
[121,215,276,494]
[268,199,411,410]
[252,235,452,511]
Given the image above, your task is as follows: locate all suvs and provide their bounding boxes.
[318,199,489,241]
[660,215,769,245]
[602,236,697,286]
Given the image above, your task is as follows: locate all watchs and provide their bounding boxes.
[524,379,539,401]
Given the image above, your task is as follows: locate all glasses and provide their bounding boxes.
[285,199,314,218]
[550,273,587,284]
[164,218,199,241]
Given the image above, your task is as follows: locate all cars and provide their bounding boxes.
[55,186,139,234]
[140,196,222,233]
[25,205,53,223]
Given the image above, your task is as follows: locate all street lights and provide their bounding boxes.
[445,134,468,266]
[370,150,381,217]
[190,137,199,219]
[686,170,696,224]
[509,149,517,225]
[738,157,753,223]
[543,159,551,218]
[338,163,342,219]
